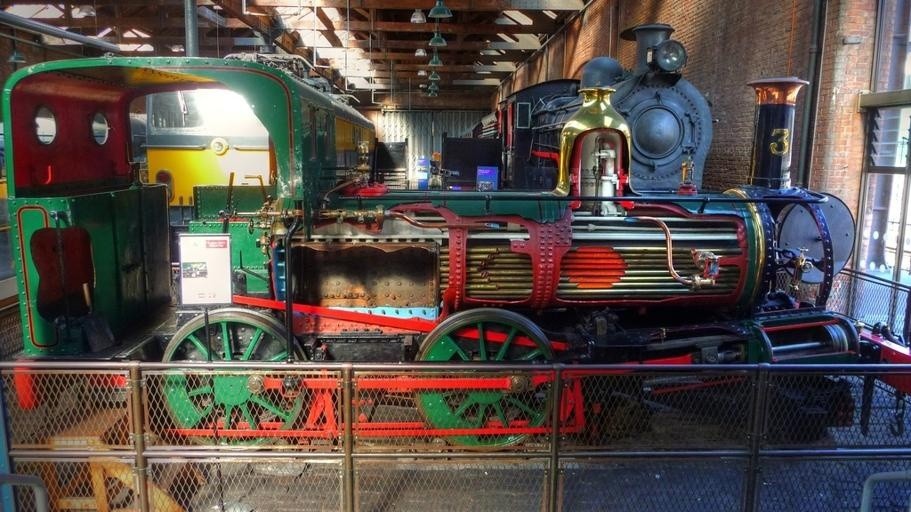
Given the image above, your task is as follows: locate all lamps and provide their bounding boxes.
[426,1,453,98]
